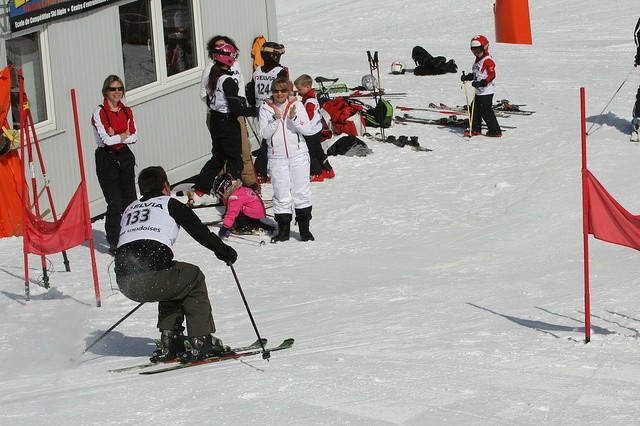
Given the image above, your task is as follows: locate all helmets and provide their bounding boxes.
[471,34,489,53]
[391,61,403,73]
[213,173,233,199]
[211,43,235,67]
[260,41,286,63]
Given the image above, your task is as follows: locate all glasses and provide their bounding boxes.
[108,86,124,91]
[214,48,239,58]
[273,89,287,93]
[470,43,490,54]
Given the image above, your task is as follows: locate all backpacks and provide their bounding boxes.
[353,99,393,128]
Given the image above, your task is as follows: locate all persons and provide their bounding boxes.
[293,73,336,182]
[411,46,458,75]
[213,172,266,235]
[195,38,253,188]
[630,17,639,142]
[113,166,238,358]
[259,78,315,243]
[460,35,503,137]
[245,40,290,180]
[200,34,260,192]
[91,75,138,257]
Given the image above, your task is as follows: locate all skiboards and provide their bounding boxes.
[631,129,639,142]
[395,113,516,132]
[187,198,276,225]
[347,92,408,99]
[105,338,294,374]
[430,102,532,118]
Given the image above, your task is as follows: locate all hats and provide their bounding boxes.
[138,167,167,196]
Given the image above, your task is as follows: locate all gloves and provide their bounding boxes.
[219,226,231,238]
[461,73,474,81]
[472,78,487,87]
[215,244,237,266]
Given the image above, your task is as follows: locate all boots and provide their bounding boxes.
[271,214,292,243]
[295,205,314,242]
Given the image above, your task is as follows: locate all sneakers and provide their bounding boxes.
[110,245,116,251]
[161,329,189,351]
[486,131,502,137]
[406,136,419,147]
[232,225,251,235]
[310,172,324,182]
[632,117,640,132]
[321,168,335,179]
[393,136,408,147]
[191,334,223,352]
[464,130,481,136]
[192,191,220,207]
